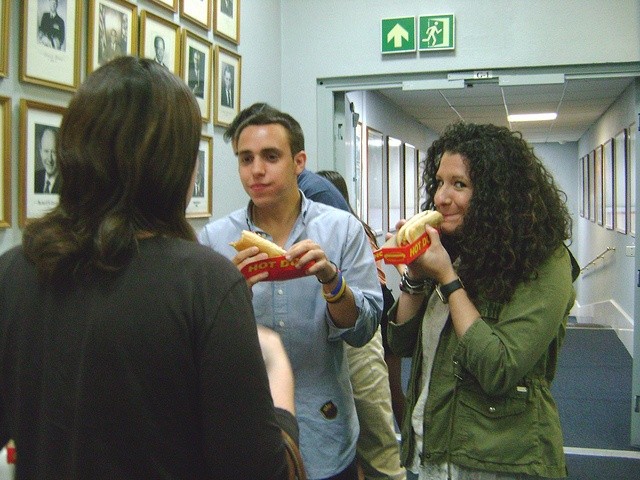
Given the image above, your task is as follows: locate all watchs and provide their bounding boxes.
[434,278,466,304]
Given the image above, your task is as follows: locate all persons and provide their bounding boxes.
[190,50,205,96]
[224,103,403,480]
[33,128,61,192]
[37,0,64,49]
[199,105,384,480]
[103,31,126,63]
[191,156,205,198]
[383,122,574,475]
[1,56,302,480]
[148,36,173,67]
[317,170,408,433]
[221,69,239,109]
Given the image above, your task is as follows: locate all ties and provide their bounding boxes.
[50,15,53,21]
[44,181,50,193]
[197,184,198,196]
[227,90,231,106]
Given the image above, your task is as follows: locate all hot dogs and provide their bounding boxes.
[228,230,288,257]
[397,210,443,248]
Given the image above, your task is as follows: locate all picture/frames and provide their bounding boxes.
[16,97,69,230]
[213,44,242,129]
[213,0,240,45]
[179,0,212,31]
[17,0,81,93]
[149,0,179,13]
[0,2,10,80]
[139,8,181,78]
[0,94,13,230]
[180,27,214,123]
[185,133,214,219]
[86,0,139,77]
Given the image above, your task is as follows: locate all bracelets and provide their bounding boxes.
[318,261,339,283]
[322,272,347,303]
[400,268,429,296]
